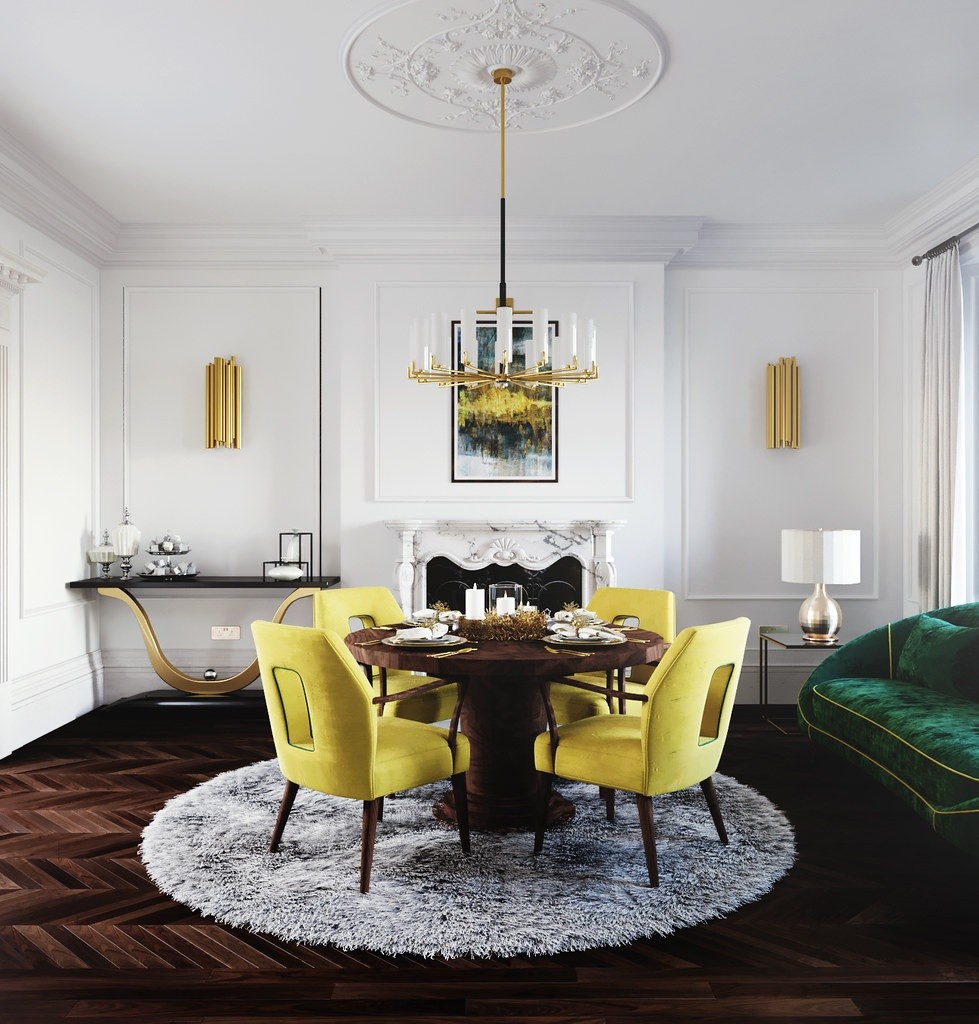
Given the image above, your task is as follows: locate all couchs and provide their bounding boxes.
[798,604,979,852]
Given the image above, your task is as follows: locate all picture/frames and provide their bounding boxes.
[450,319,559,484]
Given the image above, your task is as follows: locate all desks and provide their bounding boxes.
[344,616,664,831]
[69,575,339,696]
[759,633,844,738]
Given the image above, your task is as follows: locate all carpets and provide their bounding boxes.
[140,757,796,958]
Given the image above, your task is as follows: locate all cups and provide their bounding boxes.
[489,581,522,614]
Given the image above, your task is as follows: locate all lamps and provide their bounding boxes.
[87,529,117,578]
[112,507,140,580]
[780,527,861,643]
[406,71,598,390]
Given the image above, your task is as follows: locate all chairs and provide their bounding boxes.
[531,615,751,888]
[313,586,456,821]
[547,586,676,799]
[250,619,472,895]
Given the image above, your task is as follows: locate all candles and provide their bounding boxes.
[522,602,537,614]
[497,591,516,617]
[465,583,486,621]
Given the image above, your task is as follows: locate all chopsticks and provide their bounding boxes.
[627,638,651,643]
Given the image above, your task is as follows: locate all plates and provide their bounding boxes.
[382,634,468,647]
[401,619,432,626]
[551,623,602,634]
[543,634,628,645]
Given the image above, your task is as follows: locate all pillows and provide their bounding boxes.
[896,613,979,697]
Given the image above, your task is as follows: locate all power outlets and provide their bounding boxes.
[758,626,789,638]
[211,627,240,640]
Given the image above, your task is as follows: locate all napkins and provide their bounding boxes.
[412,609,461,620]
[551,624,622,641]
[555,610,597,620]
[394,623,448,644]
